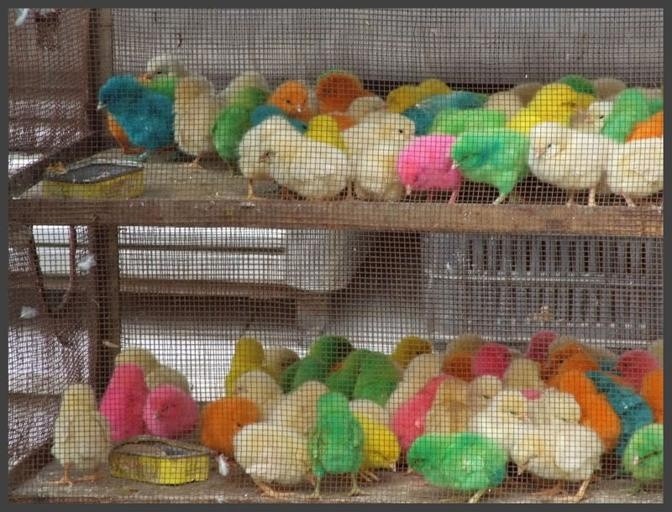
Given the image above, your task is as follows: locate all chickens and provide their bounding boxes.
[97,53,665,210]
[49,331,663,504]
